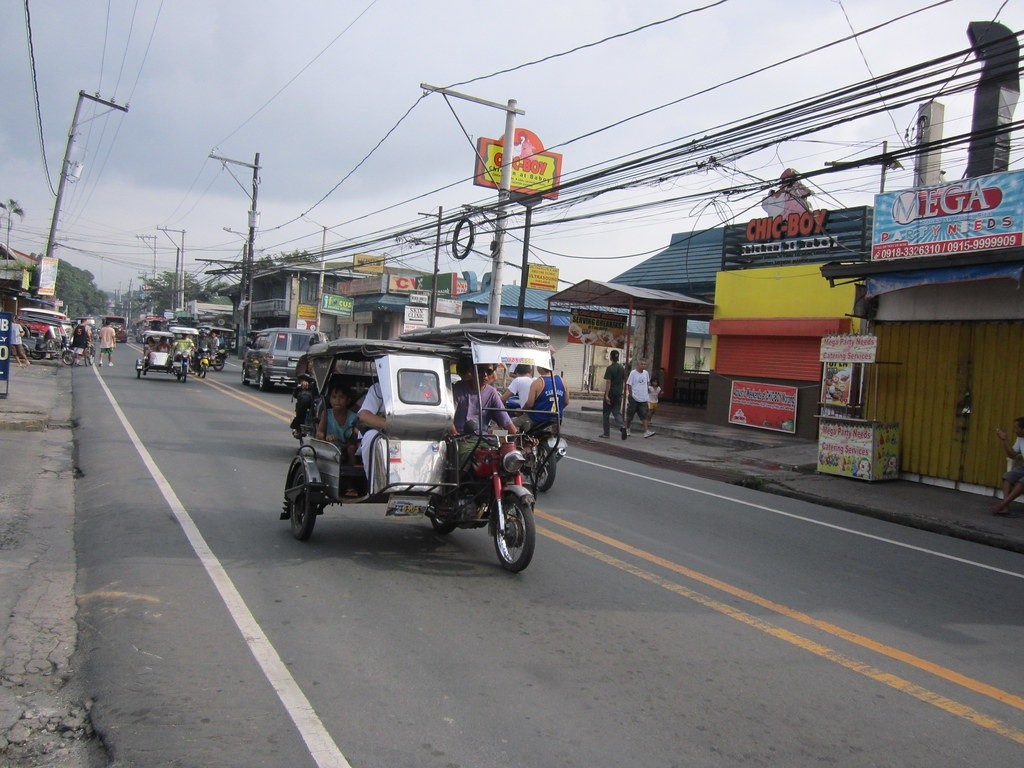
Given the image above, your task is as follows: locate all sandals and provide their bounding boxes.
[344,489,358,496]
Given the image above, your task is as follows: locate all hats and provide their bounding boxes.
[201,333,206,336]
[611,350,620,361]
[514,364,530,374]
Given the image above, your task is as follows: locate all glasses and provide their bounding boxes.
[478,367,495,375]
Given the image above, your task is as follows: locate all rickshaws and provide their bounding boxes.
[191,324,236,379]
[279,336,562,574]
[135,326,200,383]
[13,308,102,368]
[391,323,571,492]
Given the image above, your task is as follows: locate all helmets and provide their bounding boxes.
[309,332,329,345]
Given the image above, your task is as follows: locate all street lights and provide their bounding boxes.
[222,225,256,340]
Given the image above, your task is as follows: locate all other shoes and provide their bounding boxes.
[18,363,22,367]
[621,427,627,440]
[294,428,302,439]
[25,362,30,367]
[109,363,114,366]
[599,434,609,437]
[99,362,103,366]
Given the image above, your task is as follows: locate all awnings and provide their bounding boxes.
[173,310,193,324]
[26,296,55,307]
[544,278,713,419]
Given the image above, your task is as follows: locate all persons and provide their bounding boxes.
[142,327,226,372]
[598,350,627,440]
[99,317,115,366]
[625,358,656,438]
[991,416,1024,513]
[641,377,664,424]
[11,322,30,367]
[73,319,95,367]
[290,329,570,499]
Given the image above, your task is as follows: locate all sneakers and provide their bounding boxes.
[626,428,631,435]
[643,431,655,438]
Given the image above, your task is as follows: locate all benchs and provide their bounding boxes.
[314,387,367,478]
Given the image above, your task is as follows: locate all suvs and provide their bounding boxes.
[242,327,331,393]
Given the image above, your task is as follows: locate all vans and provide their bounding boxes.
[101,316,130,343]
[134,316,170,345]
